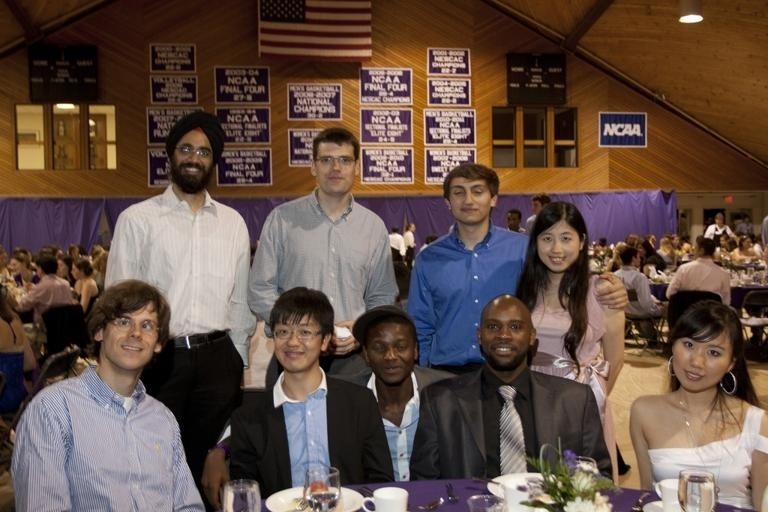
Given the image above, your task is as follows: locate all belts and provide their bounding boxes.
[165,328,232,353]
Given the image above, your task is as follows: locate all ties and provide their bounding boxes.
[494,382,532,478]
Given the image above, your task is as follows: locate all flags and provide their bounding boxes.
[259,0,373,62]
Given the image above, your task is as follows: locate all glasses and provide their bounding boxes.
[269,324,326,342]
[313,153,357,167]
[169,141,214,159]
[105,314,163,335]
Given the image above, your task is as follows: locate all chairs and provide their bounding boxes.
[626,284,768,358]
[2,347,81,453]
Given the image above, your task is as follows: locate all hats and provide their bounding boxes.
[161,107,230,167]
[351,303,419,343]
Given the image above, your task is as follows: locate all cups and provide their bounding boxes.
[469,470,721,511]
[682,249,768,289]
[646,268,675,285]
[302,466,342,512]
[582,240,616,272]
[362,487,409,512]
[222,478,262,512]
[2,278,37,300]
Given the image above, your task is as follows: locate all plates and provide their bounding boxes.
[264,485,365,512]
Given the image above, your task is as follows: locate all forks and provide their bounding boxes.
[295,498,308,511]
